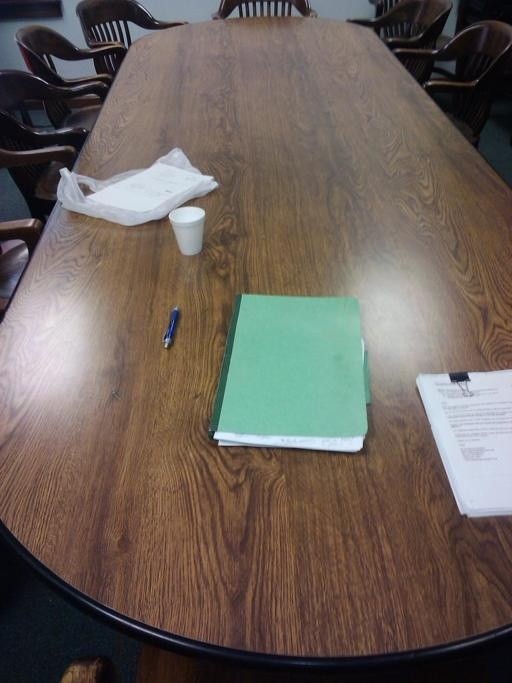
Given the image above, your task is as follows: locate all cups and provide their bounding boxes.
[169,206,205,257]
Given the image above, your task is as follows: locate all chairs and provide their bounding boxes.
[1,68,106,216]
[0,218,43,325]
[75,2,189,87]
[12,23,126,131]
[345,0,453,90]
[212,2,317,18]
[394,20,511,150]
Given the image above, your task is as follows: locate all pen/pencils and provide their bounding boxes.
[162,305,179,348]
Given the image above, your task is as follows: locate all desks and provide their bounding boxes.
[0,19,512,664]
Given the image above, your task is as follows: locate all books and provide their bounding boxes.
[85,162,217,219]
[208,292,370,454]
